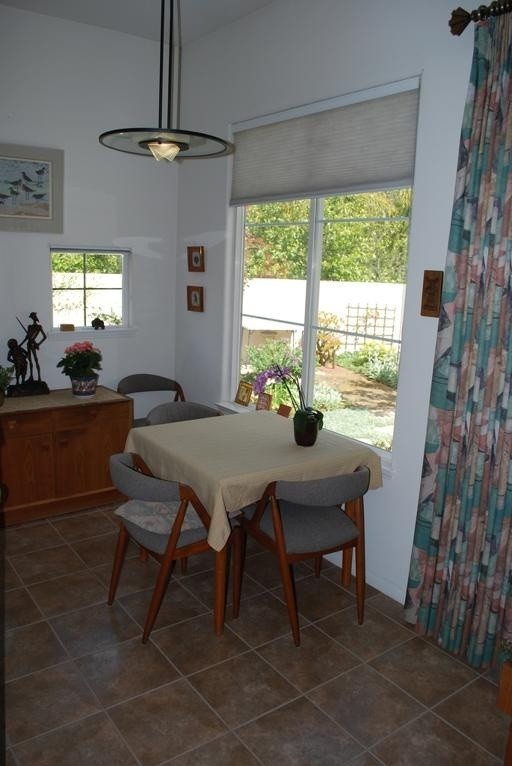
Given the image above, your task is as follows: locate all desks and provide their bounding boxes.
[120,407,382,639]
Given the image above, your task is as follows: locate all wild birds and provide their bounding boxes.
[0,166,48,202]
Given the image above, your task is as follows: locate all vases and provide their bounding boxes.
[69,375,100,398]
[293,405,318,445]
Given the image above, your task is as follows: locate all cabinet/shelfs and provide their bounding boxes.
[1,385,135,525]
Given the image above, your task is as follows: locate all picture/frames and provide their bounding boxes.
[186,284,206,313]
[420,270,444,320]
[0,140,66,236]
[234,381,253,405]
[257,393,274,412]
[186,244,207,271]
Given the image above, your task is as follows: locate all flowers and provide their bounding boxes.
[57,339,105,378]
[250,343,310,411]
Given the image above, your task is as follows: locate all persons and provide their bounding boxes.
[7,339,29,384]
[18,312,47,381]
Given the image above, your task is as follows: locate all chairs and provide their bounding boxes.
[143,398,224,425]
[106,449,228,649]
[116,375,187,411]
[215,461,378,647]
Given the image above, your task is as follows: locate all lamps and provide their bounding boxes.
[94,3,238,161]
